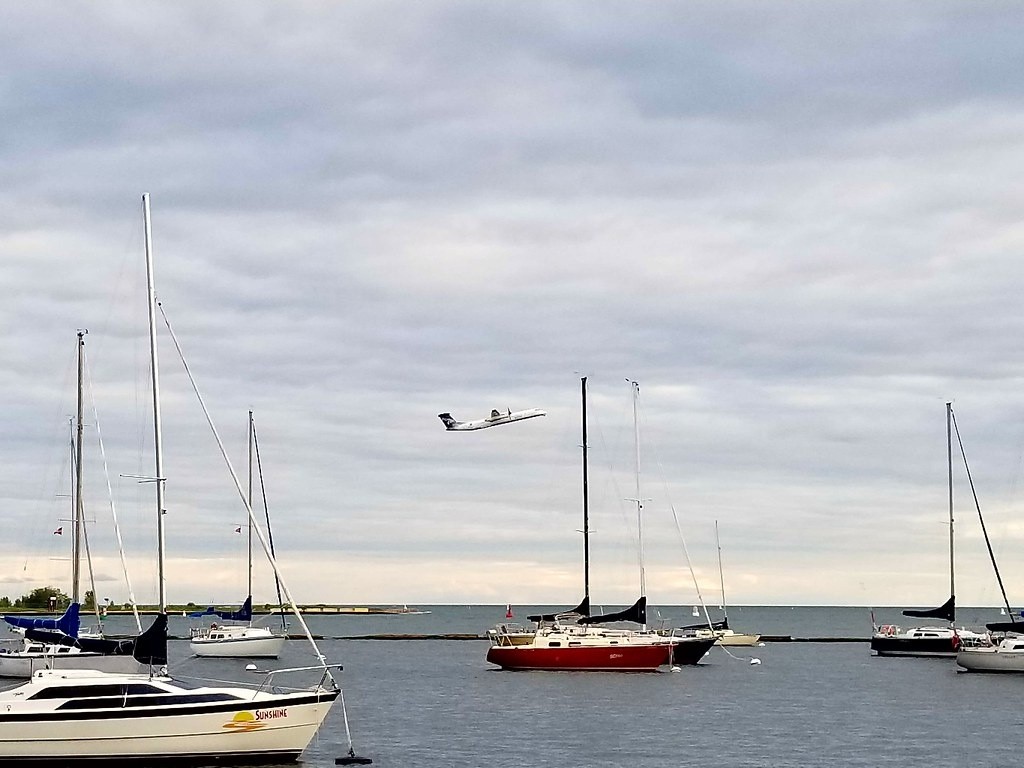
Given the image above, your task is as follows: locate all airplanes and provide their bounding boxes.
[438,407,548,431]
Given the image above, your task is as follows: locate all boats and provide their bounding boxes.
[867,607,1019,658]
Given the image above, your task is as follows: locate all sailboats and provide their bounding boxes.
[658,502,761,645]
[487,376,680,673]
[189,407,290,657]
[0,326,168,679]
[494,377,726,666]
[0,189,344,765]
[951,400,1023,672]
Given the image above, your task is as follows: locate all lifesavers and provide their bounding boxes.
[880,626,893,635]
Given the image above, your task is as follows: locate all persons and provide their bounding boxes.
[212,620,218,626]
[981,631,999,647]
